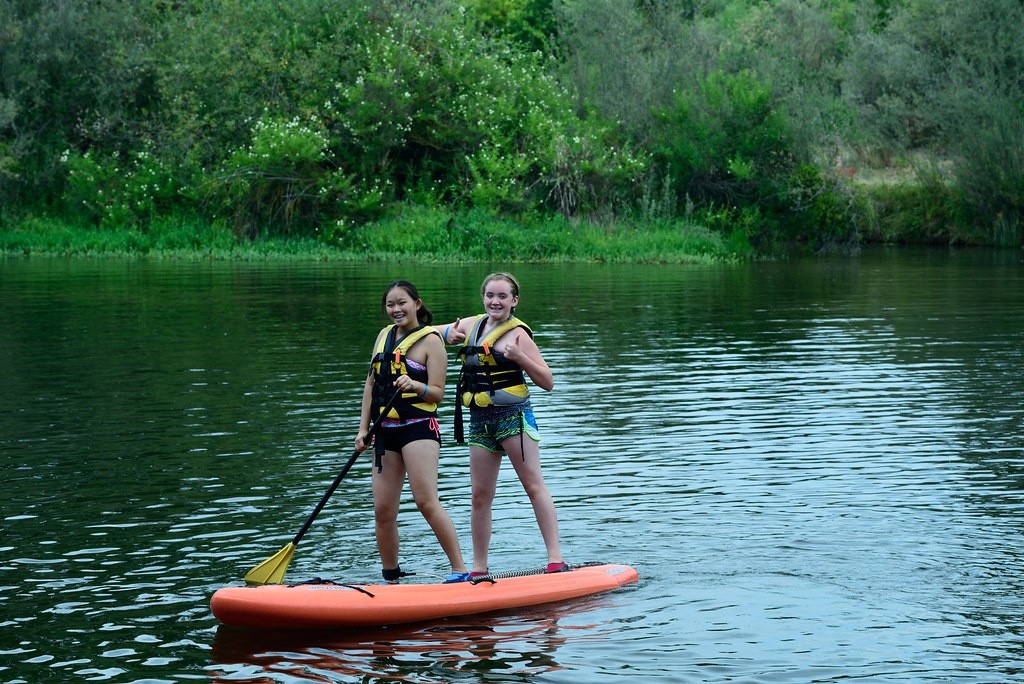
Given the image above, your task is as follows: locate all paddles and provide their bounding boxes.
[242,385,403,585]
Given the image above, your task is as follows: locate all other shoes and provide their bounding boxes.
[542,565,569,573]
[470,567,490,578]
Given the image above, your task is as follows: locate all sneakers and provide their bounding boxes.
[442,572,472,584]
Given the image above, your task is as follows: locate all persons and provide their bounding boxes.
[355,281,472,585]
[431,272,566,577]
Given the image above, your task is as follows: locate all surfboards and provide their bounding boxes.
[206,560,641,632]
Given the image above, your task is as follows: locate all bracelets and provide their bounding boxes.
[444,327,450,340]
[418,384,429,398]
